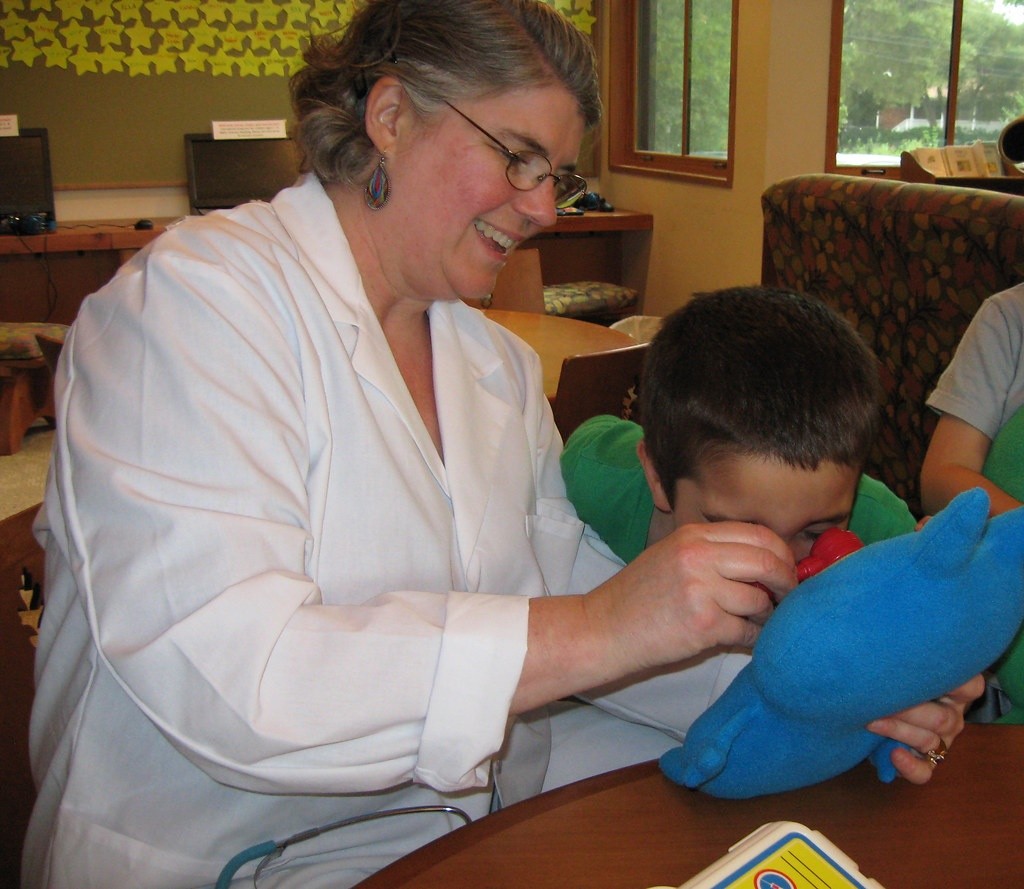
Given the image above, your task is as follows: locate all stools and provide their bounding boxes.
[0,320,70,454]
[544,279,640,324]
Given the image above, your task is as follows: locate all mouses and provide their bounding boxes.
[7,214,42,234]
[134,219,154,230]
[600,202,615,212]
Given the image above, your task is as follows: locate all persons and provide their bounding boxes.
[920,282,1024,516]
[14,0,988,889]
[557,285,920,568]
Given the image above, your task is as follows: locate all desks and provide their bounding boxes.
[347,724,1024,889]
[0,207,652,267]
[478,309,640,407]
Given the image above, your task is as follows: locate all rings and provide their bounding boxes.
[929,739,947,766]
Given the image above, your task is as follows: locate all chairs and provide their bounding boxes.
[0,501,46,889]
[461,248,544,316]
[555,342,643,444]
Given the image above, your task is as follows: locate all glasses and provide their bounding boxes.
[439,97,587,210]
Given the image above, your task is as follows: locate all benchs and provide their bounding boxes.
[760,171,1024,521]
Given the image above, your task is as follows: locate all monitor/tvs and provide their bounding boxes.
[0,128,57,222]
[184,132,299,216]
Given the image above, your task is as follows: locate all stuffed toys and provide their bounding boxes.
[655,486,1024,799]
